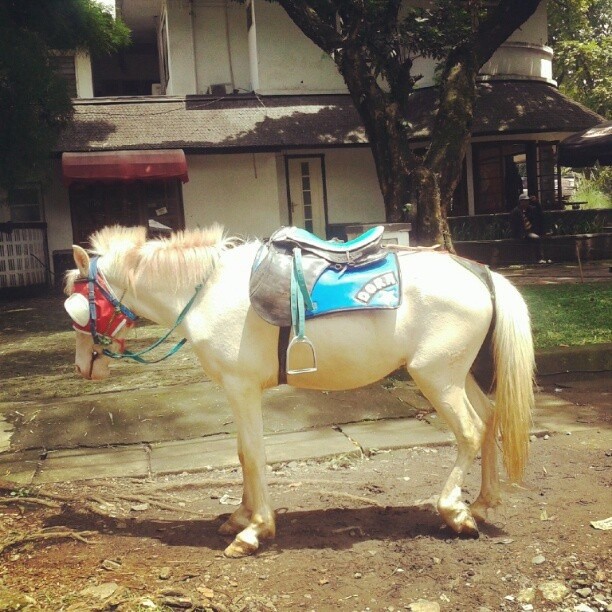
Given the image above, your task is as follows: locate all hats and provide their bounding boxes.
[519,194,530,200]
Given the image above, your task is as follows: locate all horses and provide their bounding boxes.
[62,225,533,559]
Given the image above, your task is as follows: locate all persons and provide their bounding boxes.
[510,194,553,263]
